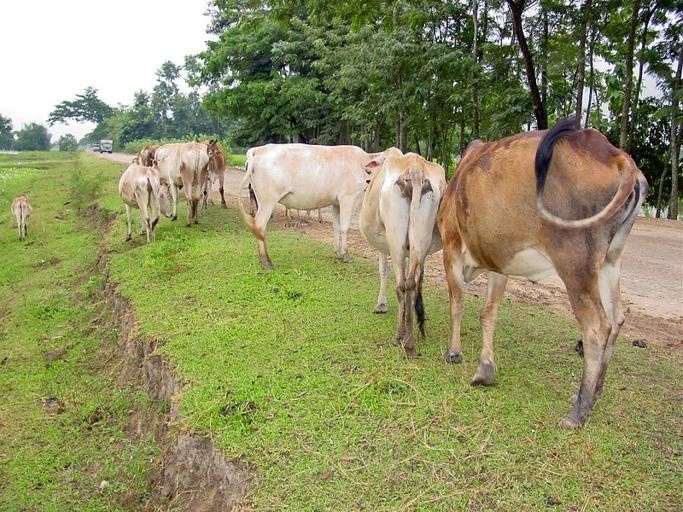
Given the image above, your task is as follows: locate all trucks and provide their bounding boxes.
[98,139,113,153]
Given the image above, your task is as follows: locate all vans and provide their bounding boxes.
[92,143,99,152]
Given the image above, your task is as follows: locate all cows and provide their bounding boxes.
[202,139,228,211]
[358,145,448,360]
[243,160,273,218]
[119,160,173,246]
[151,141,210,229]
[10,194,34,242]
[133,144,160,167]
[238,142,382,271]
[436,114,650,429]
[282,207,321,228]
